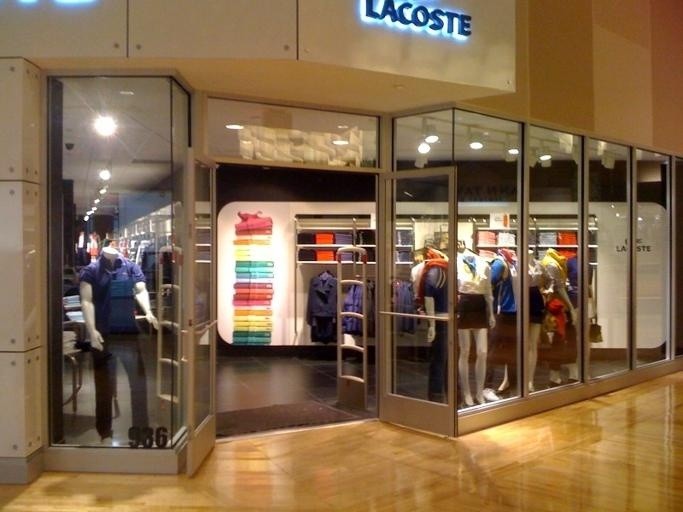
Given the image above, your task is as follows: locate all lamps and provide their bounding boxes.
[92,109,116,137]
[82,162,112,222]
[414,115,617,170]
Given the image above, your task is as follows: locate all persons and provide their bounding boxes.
[74,229,151,266]
[414,247,579,408]
[78,247,159,447]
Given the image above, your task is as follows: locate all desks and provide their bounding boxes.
[63,349,121,417]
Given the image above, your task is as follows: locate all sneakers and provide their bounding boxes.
[481,387,499,402]
[100,435,112,445]
